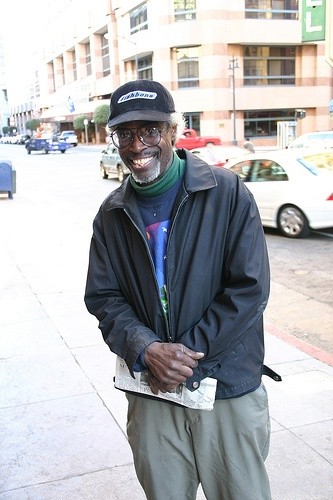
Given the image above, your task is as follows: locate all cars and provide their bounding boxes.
[175,128,221,150]
[189,145,253,168]
[0,130,79,154]
[99,141,132,183]
[286,132,333,149]
[222,149,333,239]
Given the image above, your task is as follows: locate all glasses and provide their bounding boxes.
[110,123,169,149]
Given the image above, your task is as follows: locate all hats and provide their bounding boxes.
[108,80,176,128]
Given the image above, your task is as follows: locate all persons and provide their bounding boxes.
[85,80,280,500]
